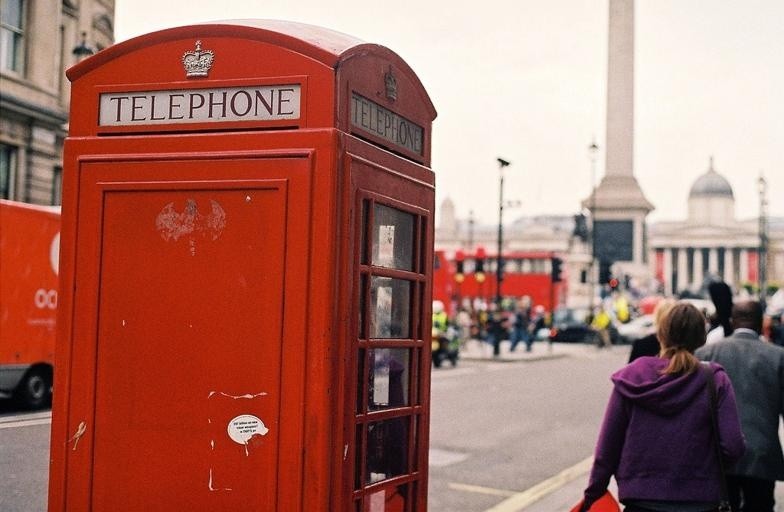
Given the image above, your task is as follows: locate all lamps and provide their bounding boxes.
[72,32,93,63]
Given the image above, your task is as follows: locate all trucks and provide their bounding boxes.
[0,199,62,413]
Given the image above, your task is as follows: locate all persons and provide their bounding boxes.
[692,300,784,512]
[431,292,547,368]
[580,300,754,510]
[584,271,784,362]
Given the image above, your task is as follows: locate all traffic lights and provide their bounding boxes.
[475,248,486,283]
[610,277,619,293]
[455,251,466,283]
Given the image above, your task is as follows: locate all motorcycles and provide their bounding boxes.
[431,321,460,367]
